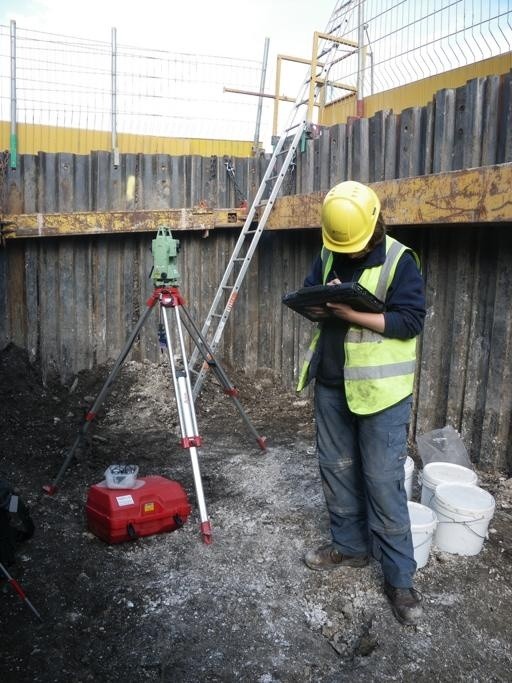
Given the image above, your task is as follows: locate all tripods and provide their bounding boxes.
[41,288,267,545]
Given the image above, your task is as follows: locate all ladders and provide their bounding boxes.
[189,0,355,403]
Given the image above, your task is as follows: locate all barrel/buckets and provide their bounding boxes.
[418,461,479,504]
[403,455,415,500]
[406,499,438,570]
[429,484,496,557]
[86,474,191,542]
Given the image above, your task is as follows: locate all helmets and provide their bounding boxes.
[321,180,381,253]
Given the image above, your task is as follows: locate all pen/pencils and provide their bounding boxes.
[332,270,339,278]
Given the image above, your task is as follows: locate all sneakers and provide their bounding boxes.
[387,585,422,625]
[304,545,369,571]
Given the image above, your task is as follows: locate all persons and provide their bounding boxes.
[294,180,430,625]
